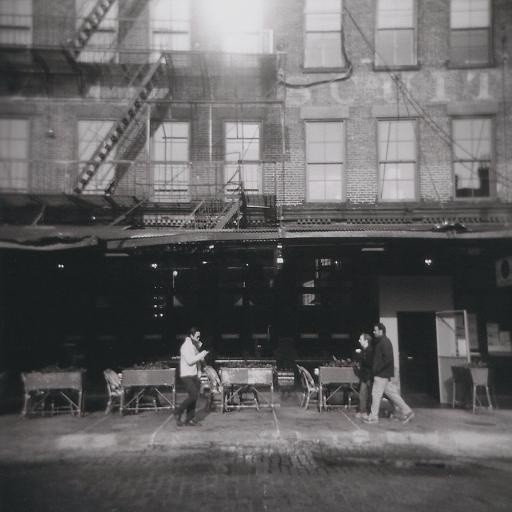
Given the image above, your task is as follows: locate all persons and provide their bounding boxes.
[363,320,415,426]
[173,327,210,427]
[352,332,401,421]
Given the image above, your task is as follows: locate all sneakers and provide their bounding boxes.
[403,413,414,424]
[357,412,378,424]
[173,412,199,427]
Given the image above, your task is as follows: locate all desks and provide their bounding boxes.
[451,363,499,412]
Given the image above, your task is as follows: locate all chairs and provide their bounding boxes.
[102,368,177,415]
[204,363,280,417]
[22,368,89,418]
[295,362,360,415]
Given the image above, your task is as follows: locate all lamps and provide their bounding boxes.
[45,128,56,137]
[275,254,285,269]
